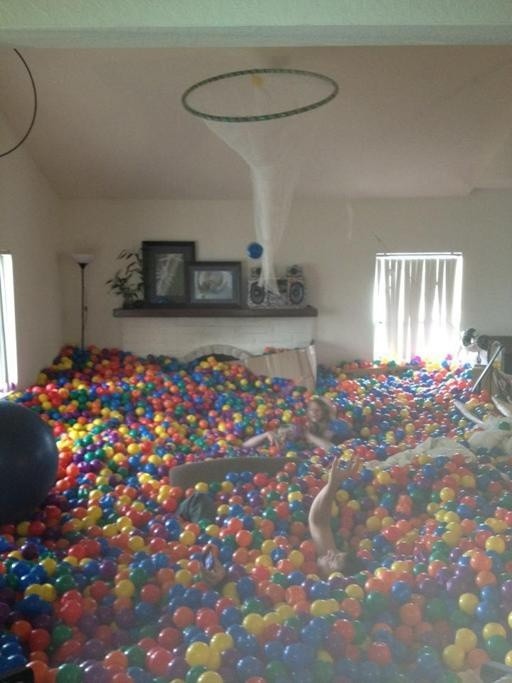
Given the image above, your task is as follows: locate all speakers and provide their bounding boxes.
[286,264,302,278]
[251,265,261,278]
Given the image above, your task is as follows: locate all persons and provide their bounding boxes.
[243,398,336,448]
[309,454,359,578]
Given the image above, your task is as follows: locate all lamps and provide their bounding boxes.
[72,253,96,352]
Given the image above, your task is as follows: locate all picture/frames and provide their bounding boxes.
[184,260,241,309]
[141,240,197,306]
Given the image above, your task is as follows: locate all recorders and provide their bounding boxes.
[247,278,307,310]
